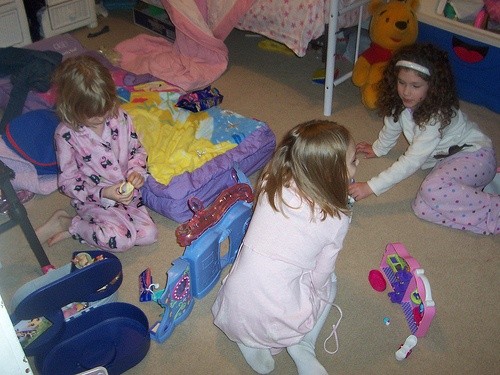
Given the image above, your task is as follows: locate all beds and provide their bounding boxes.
[142,0,371,117]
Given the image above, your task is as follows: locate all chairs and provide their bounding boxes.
[0,160,55,273]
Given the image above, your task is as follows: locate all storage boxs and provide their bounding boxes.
[133,3,175,40]
[416,0,500,114]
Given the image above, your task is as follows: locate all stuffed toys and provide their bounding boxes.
[352,0,419,110]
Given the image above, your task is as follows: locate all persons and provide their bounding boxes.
[210,119,359,375]
[347,43,500,237]
[35,55,158,253]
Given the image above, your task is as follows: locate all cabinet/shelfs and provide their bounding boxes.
[0,0,97,49]
